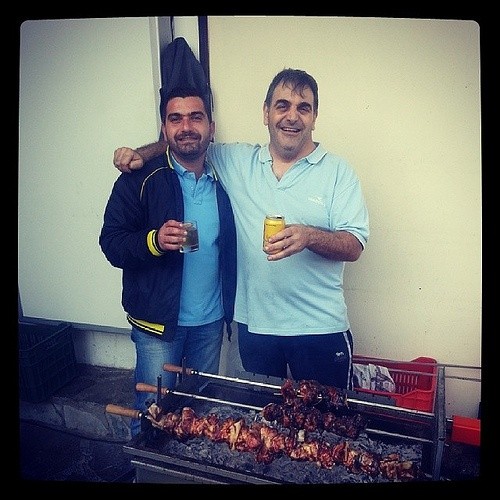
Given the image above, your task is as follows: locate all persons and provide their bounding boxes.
[98,85,237,409]
[113,68,370,390]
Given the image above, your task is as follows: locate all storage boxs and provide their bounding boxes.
[349,355,438,413]
[19,317,81,403]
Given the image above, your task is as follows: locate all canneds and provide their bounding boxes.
[263,214,285,255]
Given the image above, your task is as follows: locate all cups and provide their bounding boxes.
[179,220,199,253]
